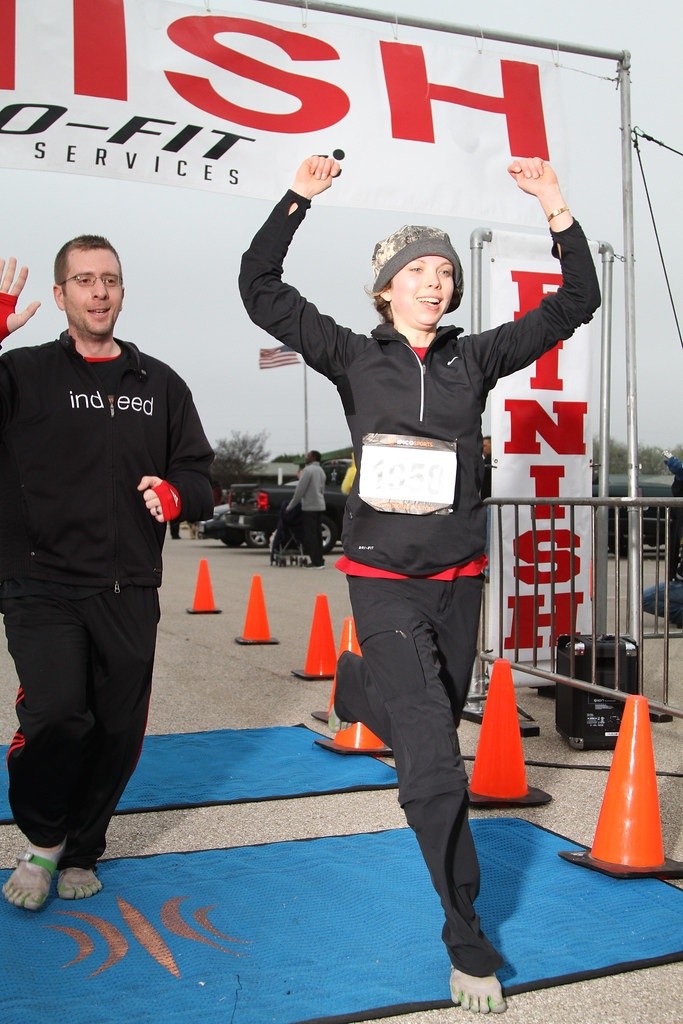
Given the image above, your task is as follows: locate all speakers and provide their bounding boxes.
[557,634,640,750]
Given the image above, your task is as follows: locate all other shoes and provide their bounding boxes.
[172,535,180,539]
[303,563,324,570]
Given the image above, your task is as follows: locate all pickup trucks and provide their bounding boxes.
[225,459,353,553]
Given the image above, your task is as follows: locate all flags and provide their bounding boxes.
[258,344,301,369]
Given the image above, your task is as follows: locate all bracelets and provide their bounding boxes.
[547,206,570,222]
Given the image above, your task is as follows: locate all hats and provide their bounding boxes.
[371,225,464,314]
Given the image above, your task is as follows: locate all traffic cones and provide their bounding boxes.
[558,695,683,880]
[291,594,338,680]
[466,657,552,807]
[311,618,393,757]
[236,574,280,645]
[186,558,222,614]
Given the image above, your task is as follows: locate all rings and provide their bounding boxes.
[155,506,160,515]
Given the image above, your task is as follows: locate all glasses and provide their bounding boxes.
[57,274,123,288]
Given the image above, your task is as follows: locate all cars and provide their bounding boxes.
[592,474,673,554]
[199,481,301,548]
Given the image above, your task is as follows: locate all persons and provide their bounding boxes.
[288,450,326,569]
[482,435,492,499]
[256,487,268,511]
[0,236,215,911]
[239,158,603,1013]
[341,452,356,496]
